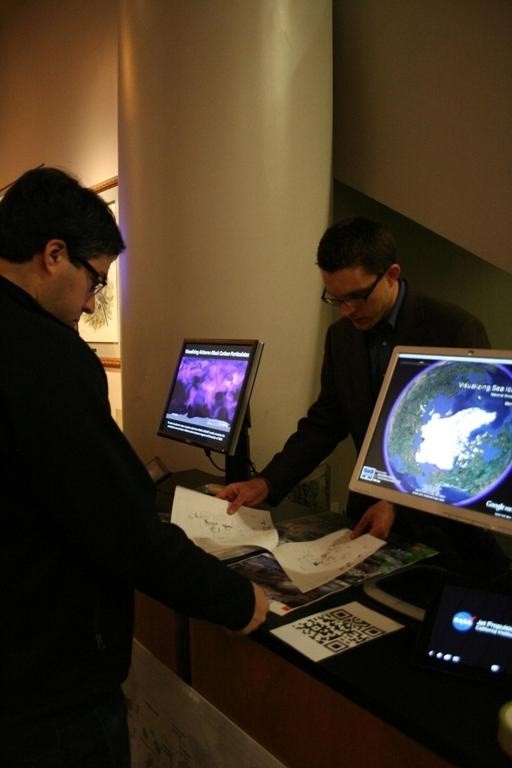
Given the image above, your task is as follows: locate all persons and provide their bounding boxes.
[0,166,270,768]
[210,218,492,539]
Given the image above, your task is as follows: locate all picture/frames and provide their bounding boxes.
[72,175,122,369]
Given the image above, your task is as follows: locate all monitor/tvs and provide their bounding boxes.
[347,344,512,621]
[155,337,265,494]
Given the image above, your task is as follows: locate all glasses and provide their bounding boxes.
[320,265,389,307]
[64,246,107,297]
